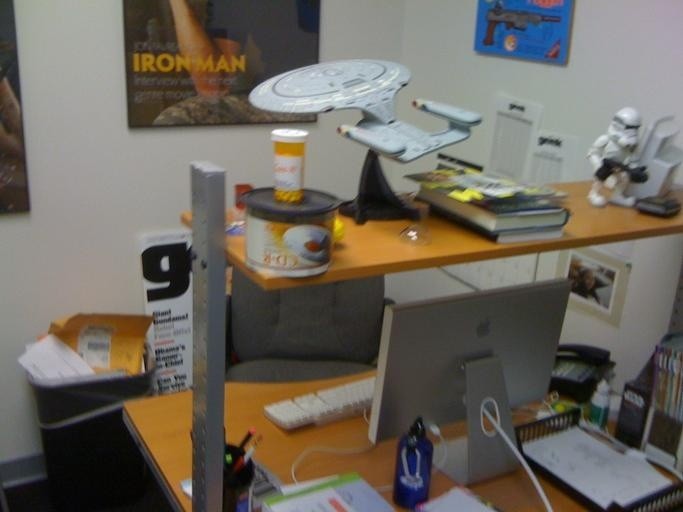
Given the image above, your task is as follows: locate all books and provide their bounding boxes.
[264,474,396,512]
[402,170,571,246]
[657,335,683,418]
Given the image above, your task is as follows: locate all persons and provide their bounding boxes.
[0,76,25,163]
[586,108,648,209]
[570,269,601,304]
[154,1,319,125]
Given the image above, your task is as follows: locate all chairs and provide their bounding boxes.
[224,265,395,381]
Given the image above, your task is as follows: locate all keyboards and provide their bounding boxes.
[262,376,376,432]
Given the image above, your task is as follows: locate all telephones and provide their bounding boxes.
[548,342,617,406]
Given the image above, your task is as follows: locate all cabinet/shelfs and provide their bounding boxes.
[121,160,682,510]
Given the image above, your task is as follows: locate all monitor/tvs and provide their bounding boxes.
[368,277,573,487]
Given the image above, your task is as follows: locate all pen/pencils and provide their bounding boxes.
[232,434,263,477]
[236,426,256,460]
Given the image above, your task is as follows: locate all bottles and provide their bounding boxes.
[391,418,433,511]
[587,379,611,432]
[272,129,307,205]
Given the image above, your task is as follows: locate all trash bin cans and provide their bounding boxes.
[23,339,157,511]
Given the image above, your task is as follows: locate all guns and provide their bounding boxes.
[482,1,562,47]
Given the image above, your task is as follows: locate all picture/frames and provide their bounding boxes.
[121,1,323,127]
[0,1,29,215]
[556,245,629,327]
[474,0,574,66]
